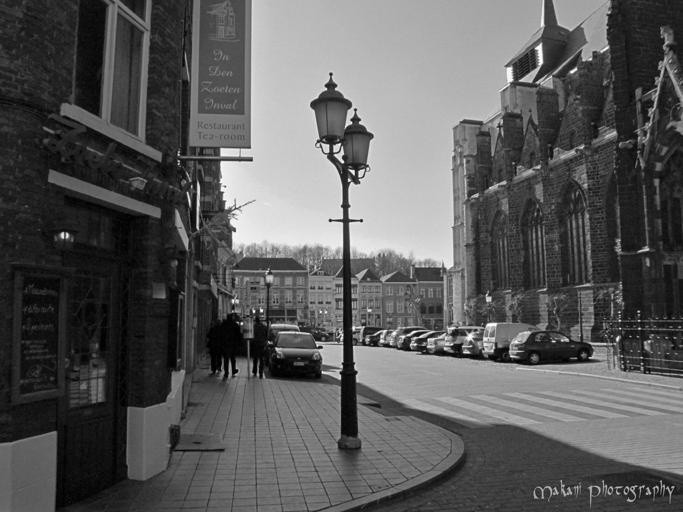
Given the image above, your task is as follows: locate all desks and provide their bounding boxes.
[263,268,273,337]
[319,308,327,324]
[310,70,374,452]
[485,290,493,323]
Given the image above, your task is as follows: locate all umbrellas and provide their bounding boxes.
[211,367,263,379]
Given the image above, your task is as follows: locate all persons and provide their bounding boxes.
[206,312,268,379]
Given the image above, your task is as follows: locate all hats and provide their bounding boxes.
[308,329,329,342]
[339,323,592,365]
[264,323,301,366]
[269,331,322,378]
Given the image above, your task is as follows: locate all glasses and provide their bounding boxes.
[45,217,82,264]
